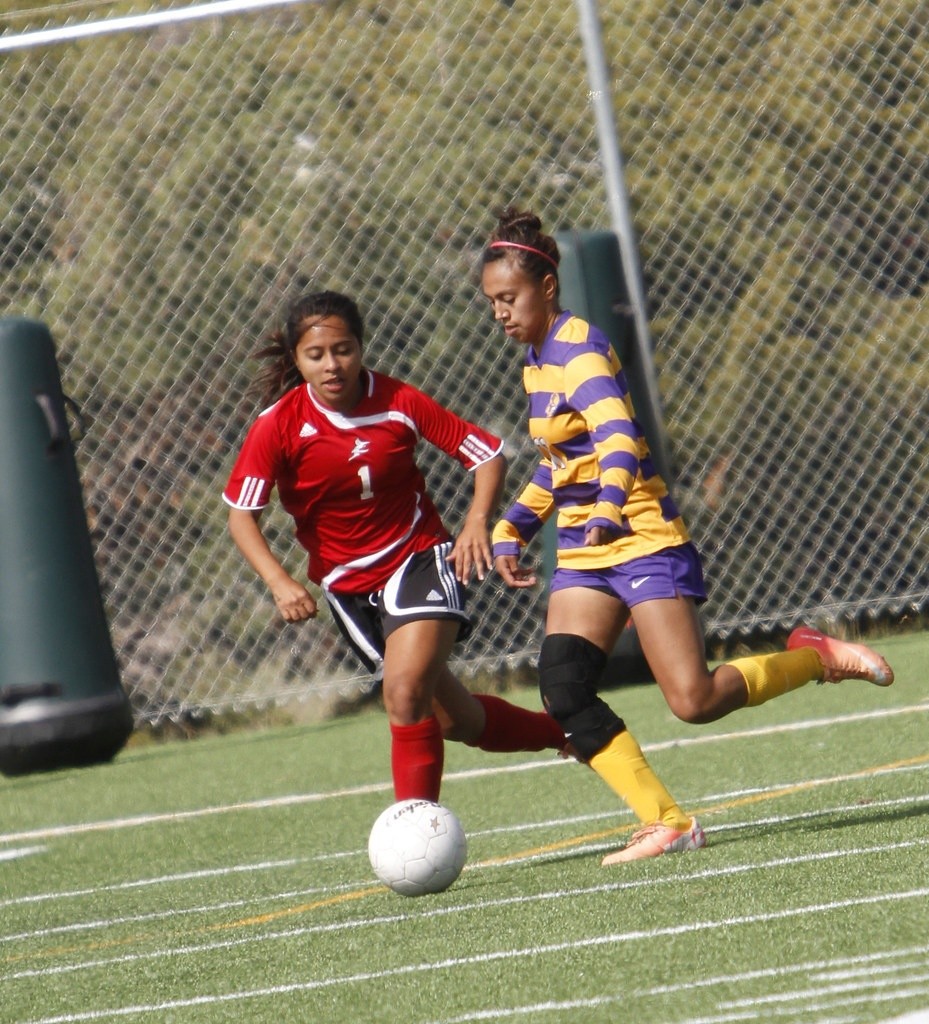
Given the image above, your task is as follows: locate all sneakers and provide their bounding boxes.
[602,816,705,866]
[542,710,585,764]
[787,627,894,687]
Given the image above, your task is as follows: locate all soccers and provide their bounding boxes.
[367,798,468,898]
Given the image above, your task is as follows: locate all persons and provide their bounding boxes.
[482,204,894,866]
[220,290,590,804]
